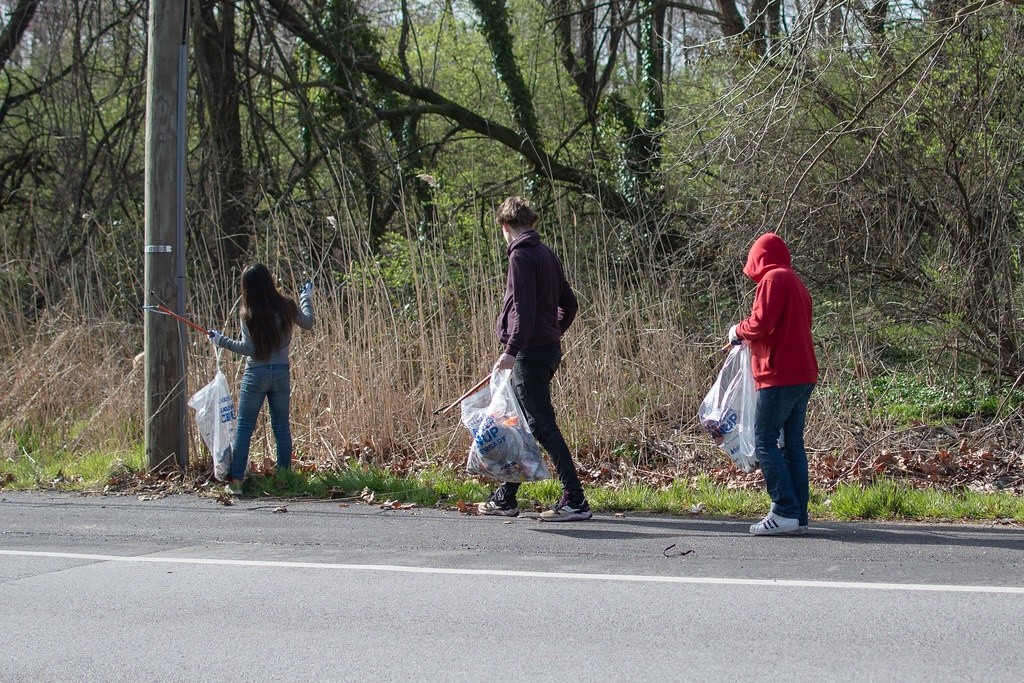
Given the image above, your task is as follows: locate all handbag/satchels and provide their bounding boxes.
[460,364,551,484]
[699,340,783,473]
[187,349,251,481]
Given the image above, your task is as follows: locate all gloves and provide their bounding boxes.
[298,280,313,299]
[207,329,222,347]
[728,325,739,341]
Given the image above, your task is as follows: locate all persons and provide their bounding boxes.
[476,200,593,522]
[729,232,819,535]
[207,263,315,496]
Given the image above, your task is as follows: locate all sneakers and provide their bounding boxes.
[750,513,799,535]
[478,498,519,517]
[783,525,809,535]
[541,491,595,520]
[224,482,243,494]
